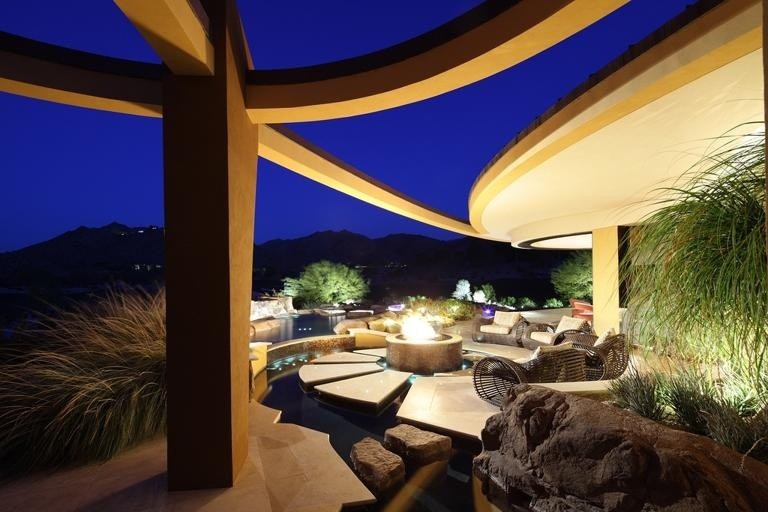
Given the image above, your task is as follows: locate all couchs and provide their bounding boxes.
[473,318,629,407]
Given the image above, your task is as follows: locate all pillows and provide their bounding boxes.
[494,311,521,327]
